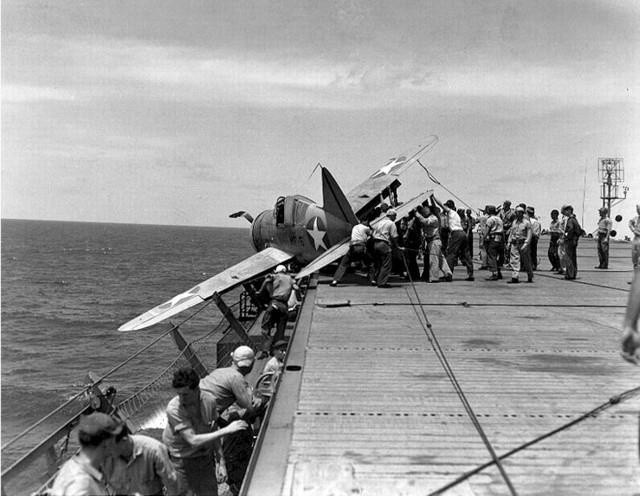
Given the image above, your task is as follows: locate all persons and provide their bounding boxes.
[263,340,287,373]
[98,421,183,496]
[255,265,299,360]
[595,207,612,269]
[626,205,640,284]
[198,344,264,424]
[618,258,640,366]
[50,412,124,496]
[161,366,249,496]
[328,199,582,287]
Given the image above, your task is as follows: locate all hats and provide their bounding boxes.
[233,345,255,367]
[79,411,123,435]
[273,341,289,349]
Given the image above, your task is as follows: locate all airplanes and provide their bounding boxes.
[118,132,445,338]
